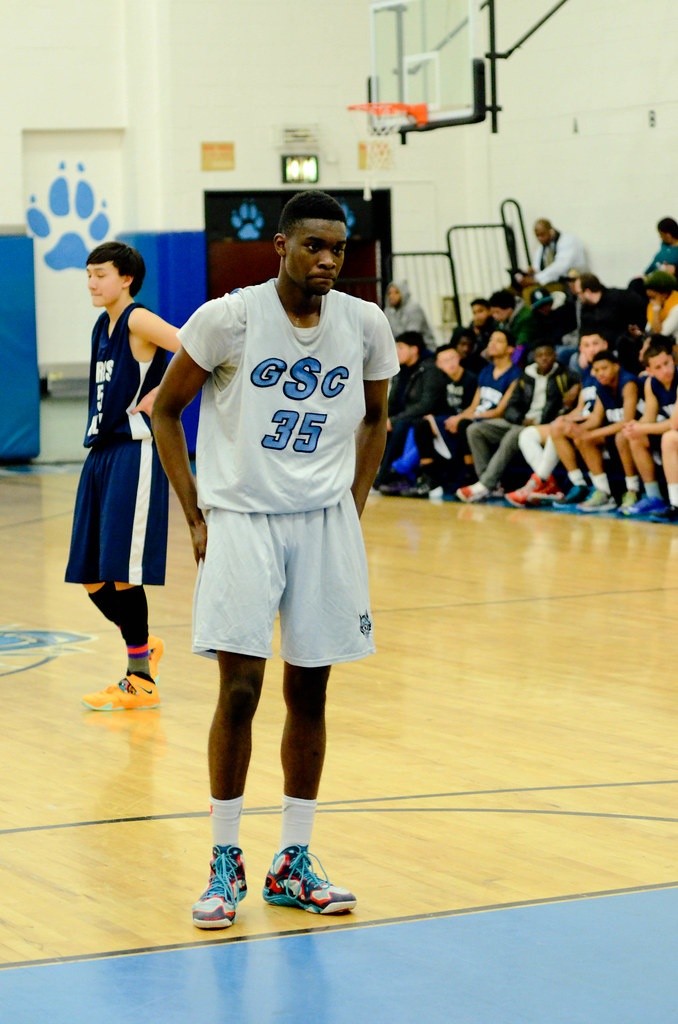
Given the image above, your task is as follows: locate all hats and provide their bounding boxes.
[645,271,675,296]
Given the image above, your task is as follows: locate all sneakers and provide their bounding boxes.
[456,479,504,503]
[617,492,637,516]
[192,843,248,928]
[627,497,664,515]
[649,504,678,525]
[148,635,168,685]
[553,484,595,509]
[80,673,160,711]
[262,843,357,914]
[576,490,617,512]
[504,473,564,509]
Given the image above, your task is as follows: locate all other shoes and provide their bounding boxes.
[380,478,413,496]
[409,485,430,499]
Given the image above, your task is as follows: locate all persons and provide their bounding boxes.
[149,191,401,934]
[65,241,182,710]
[372,216,678,524]
